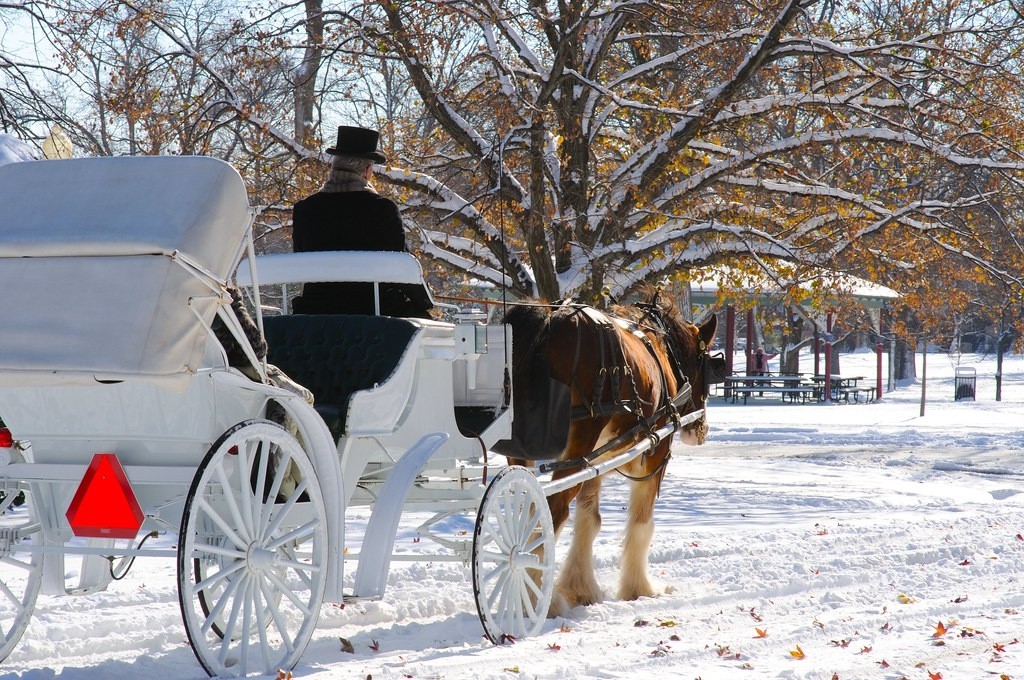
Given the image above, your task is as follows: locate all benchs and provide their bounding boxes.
[715,383,876,405]
[0,155,464,461]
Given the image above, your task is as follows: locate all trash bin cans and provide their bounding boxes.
[956,367,976,401]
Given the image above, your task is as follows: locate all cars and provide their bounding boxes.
[709,337,724,351]
[734,338,755,350]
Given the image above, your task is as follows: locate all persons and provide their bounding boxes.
[209,272,314,507]
[743,347,778,397]
[290,125,443,324]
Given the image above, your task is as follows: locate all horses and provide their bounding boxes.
[500,283,718,618]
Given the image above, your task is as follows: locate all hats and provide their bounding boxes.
[326,126,386,164]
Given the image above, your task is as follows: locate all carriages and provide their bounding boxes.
[0,156,727,679]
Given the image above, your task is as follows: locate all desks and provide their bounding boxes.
[810,374,868,401]
[724,375,809,404]
